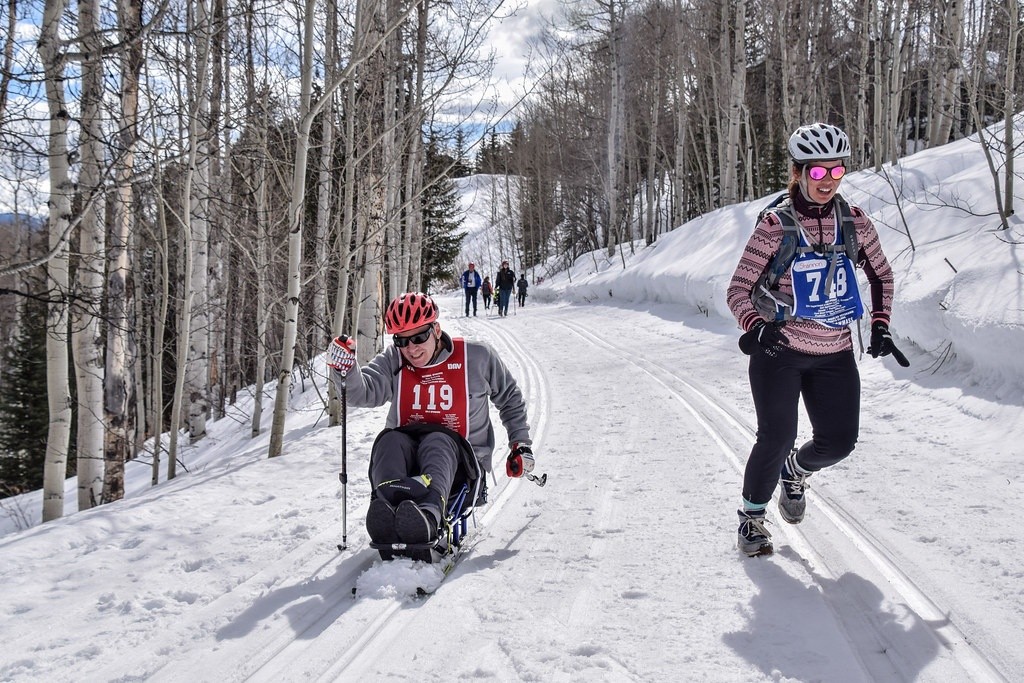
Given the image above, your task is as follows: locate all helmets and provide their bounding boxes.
[468,262,475,268]
[502,261,509,266]
[788,122,853,165]
[385,291,440,335]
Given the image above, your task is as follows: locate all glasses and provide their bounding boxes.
[392,327,433,347]
[799,160,847,181]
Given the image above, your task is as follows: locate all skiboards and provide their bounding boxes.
[349,524,489,600]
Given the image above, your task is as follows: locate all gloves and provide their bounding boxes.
[867,321,893,358]
[747,318,789,360]
[506,442,535,479]
[325,336,357,371]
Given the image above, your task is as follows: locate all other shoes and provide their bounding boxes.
[394,500,440,565]
[522,304,525,307]
[366,499,402,561]
[504,310,507,316]
[466,313,469,317]
[473,311,476,316]
[498,311,503,316]
[519,304,521,307]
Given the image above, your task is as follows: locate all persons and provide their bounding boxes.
[460,259,529,318]
[728,124,911,557]
[327,293,535,567]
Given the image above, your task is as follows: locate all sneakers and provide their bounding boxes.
[778,448,813,525]
[736,507,773,558]
